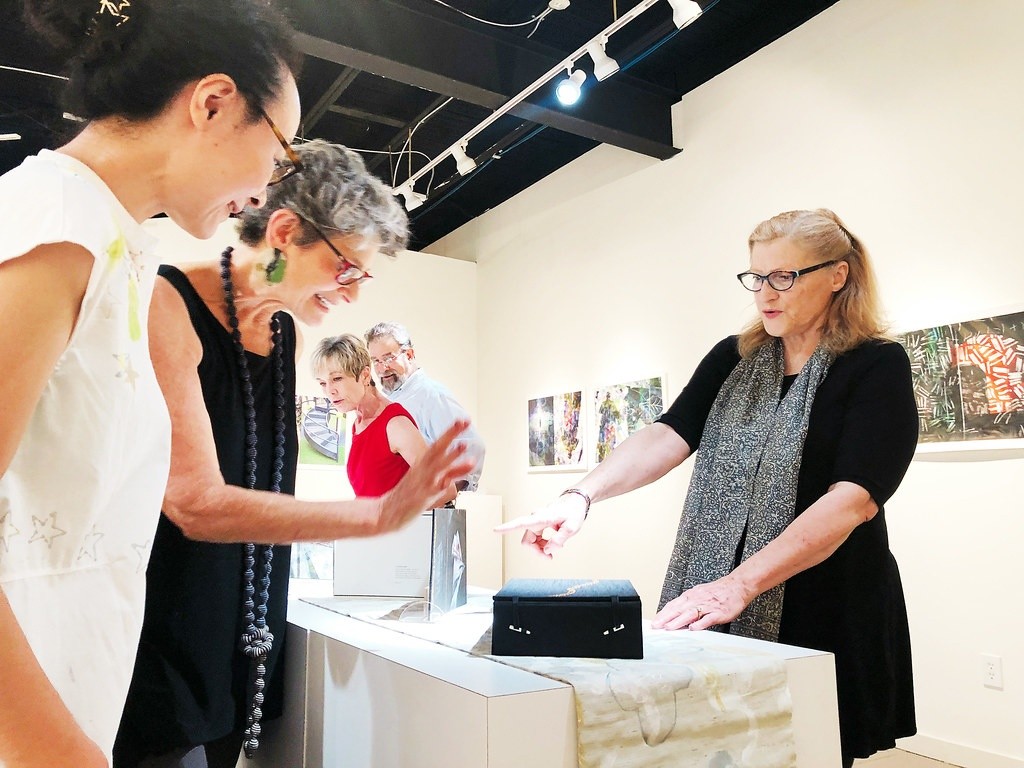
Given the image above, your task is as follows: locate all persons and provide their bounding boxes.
[311,333,458,511]
[365,321,485,510]
[111,135,409,767]
[0,0,475,768]
[494,205,918,768]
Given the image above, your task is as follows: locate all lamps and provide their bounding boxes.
[394,181,425,211]
[587,37,621,82]
[555,63,588,106]
[450,145,477,176]
[666,0,705,30]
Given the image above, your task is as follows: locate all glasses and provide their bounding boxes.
[233,84,304,187]
[737,259,839,292]
[369,348,406,368]
[294,213,373,288]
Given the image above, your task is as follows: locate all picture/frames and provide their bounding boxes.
[525,372,671,474]
[890,305,1024,454]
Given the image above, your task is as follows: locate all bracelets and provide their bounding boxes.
[561,488,591,521]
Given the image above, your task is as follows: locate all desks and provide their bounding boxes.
[239,579,842,767]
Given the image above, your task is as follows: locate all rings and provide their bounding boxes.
[692,606,701,621]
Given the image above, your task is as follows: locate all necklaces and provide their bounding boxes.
[221,247,287,756]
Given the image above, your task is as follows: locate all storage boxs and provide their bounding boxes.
[492,576,644,662]
